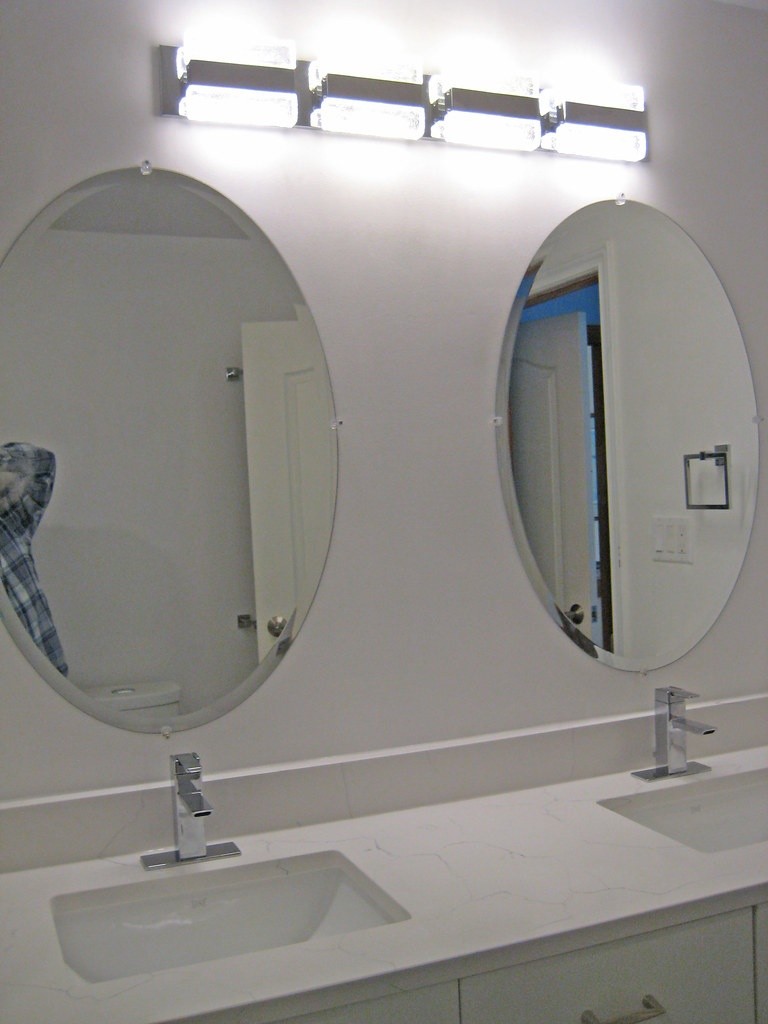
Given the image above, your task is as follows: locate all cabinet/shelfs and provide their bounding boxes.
[156,879,768,1024]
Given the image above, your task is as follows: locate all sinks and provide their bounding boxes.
[593,762,768,854]
[48,844,415,982]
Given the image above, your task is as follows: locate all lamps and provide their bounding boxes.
[157,44,647,161]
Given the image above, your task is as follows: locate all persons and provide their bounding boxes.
[0,440,69,677]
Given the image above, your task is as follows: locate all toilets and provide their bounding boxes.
[80,678,185,725]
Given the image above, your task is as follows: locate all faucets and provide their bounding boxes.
[650,685,716,776]
[170,750,217,862]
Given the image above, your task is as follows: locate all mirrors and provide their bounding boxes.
[494,198,759,673]
[0,168,339,735]
